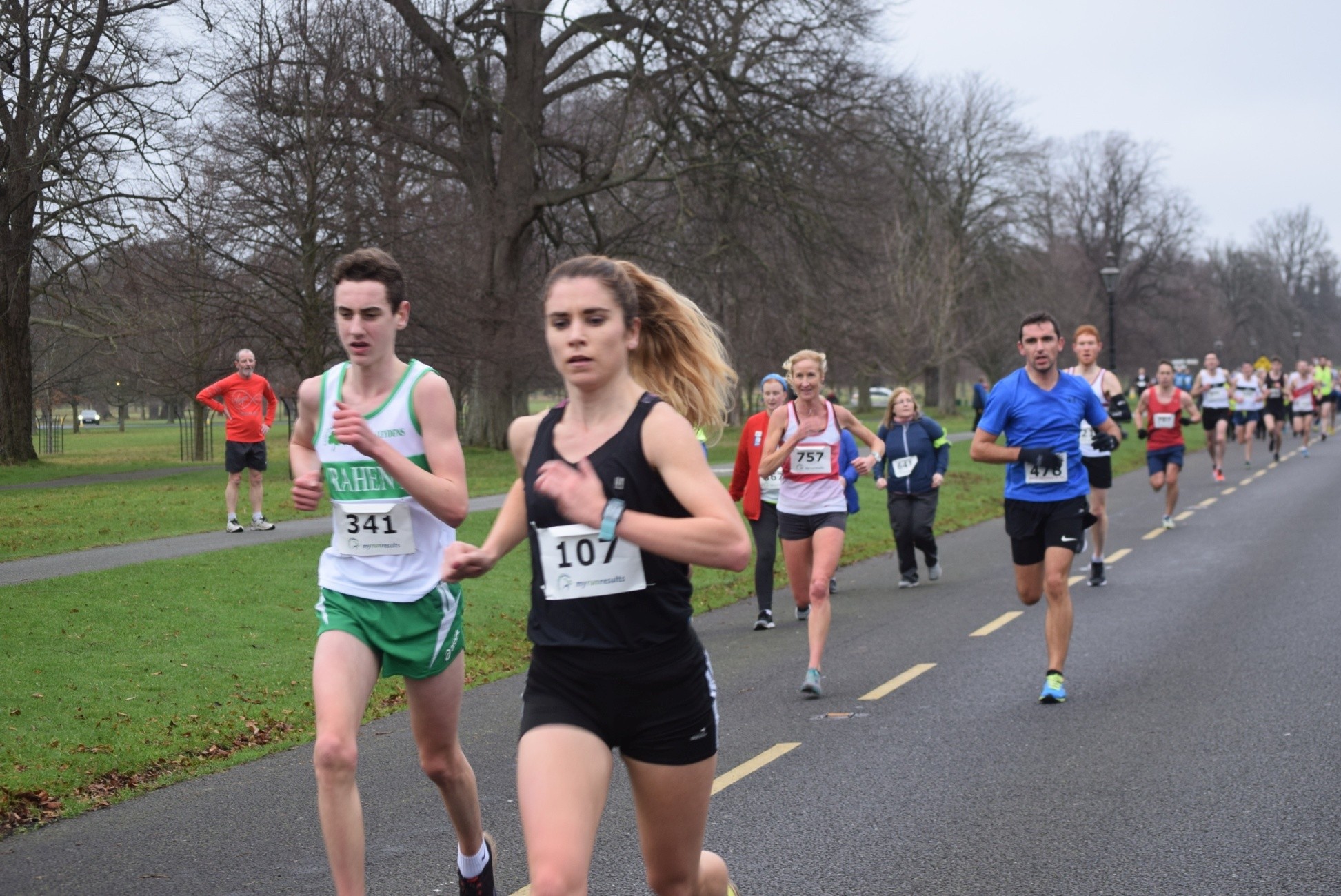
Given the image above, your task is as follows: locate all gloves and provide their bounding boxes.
[1018,446,1063,472]
[1092,433,1119,451]
[1139,429,1147,439]
[1179,418,1193,426]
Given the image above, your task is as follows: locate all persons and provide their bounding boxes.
[1190,350,1235,480]
[436,252,752,896]
[755,350,887,696]
[285,246,501,896]
[729,373,814,631]
[1060,324,1132,587]
[1128,367,1152,419]
[1134,361,1202,532]
[194,348,281,532]
[873,388,951,588]
[815,394,861,594]
[971,378,989,432]
[1172,357,1341,465]
[969,310,1122,703]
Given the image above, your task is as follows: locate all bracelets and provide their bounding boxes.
[870,451,882,463]
[599,497,625,541]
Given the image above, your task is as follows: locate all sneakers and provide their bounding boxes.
[928,562,941,580]
[800,668,826,695]
[1073,529,1088,553]
[1087,562,1107,585]
[1162,516,1175,529]
[753,611,775,629]
[251,515,276,531]
[829,580,837,594]
[795,604,811,620]
[1038,674,1067,702]
[899,573,920,588]
[457,831,497,896]
[1208,427,1336,481]
[226,518,244,533]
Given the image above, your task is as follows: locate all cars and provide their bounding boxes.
[78,410,101,426]
[852,388,895,407]
